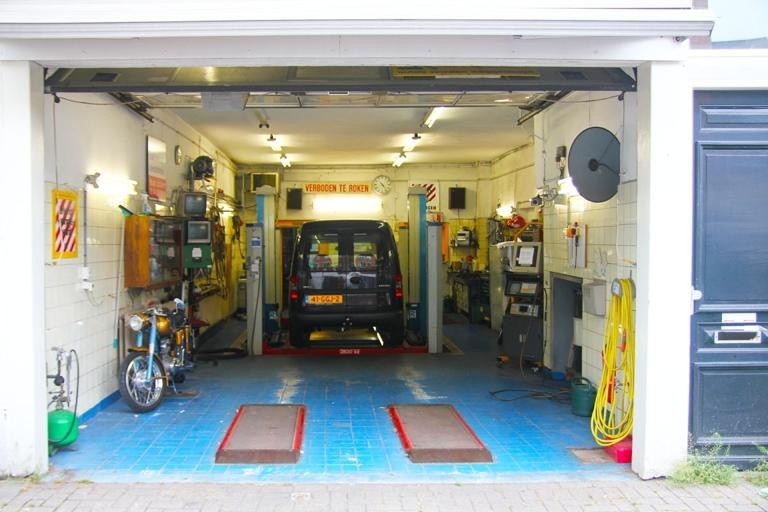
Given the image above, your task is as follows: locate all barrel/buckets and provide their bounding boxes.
[571,377,597,417]
[472,256,479,272]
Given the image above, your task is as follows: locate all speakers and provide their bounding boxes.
[449,188,465,208]
[288,189,301,209]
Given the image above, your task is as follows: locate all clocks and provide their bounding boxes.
[372,173,393,195]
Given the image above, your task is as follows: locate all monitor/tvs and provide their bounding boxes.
[187,220,211,243]
[512,242,543,274]
[179,192,208,218]
[156,221,176,243]
[251,173,278,194]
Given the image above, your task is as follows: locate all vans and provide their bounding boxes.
[282,217,405,348]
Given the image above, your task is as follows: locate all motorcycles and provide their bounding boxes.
[119,296,194,414]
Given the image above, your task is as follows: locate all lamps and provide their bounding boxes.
[391,105,444,172]
[252,107,293,168]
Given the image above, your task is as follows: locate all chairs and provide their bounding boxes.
[312,251,377,270]
